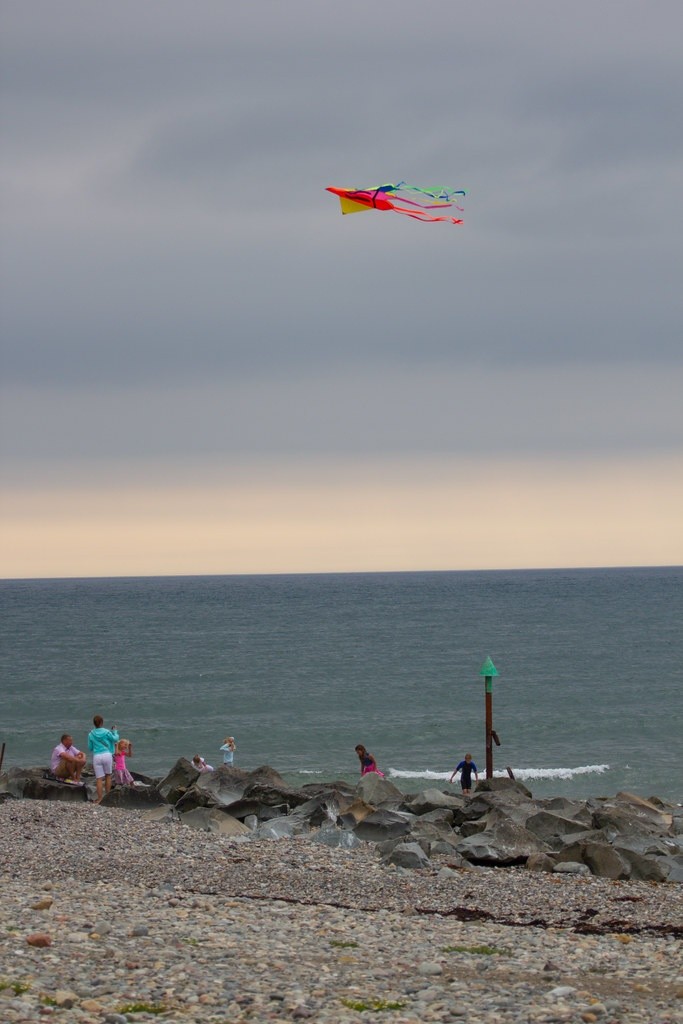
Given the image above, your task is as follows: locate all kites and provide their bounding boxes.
[326,180,468,225]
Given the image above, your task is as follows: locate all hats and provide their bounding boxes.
[465,754,471,764]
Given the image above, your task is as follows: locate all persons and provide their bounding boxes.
[220,737,236,767]
[50,735,87,782]
[88,715,119,803]
[355,744,384,777]
[191,754,213,773]
[449,754,478,794]
[115,739,135,787]
